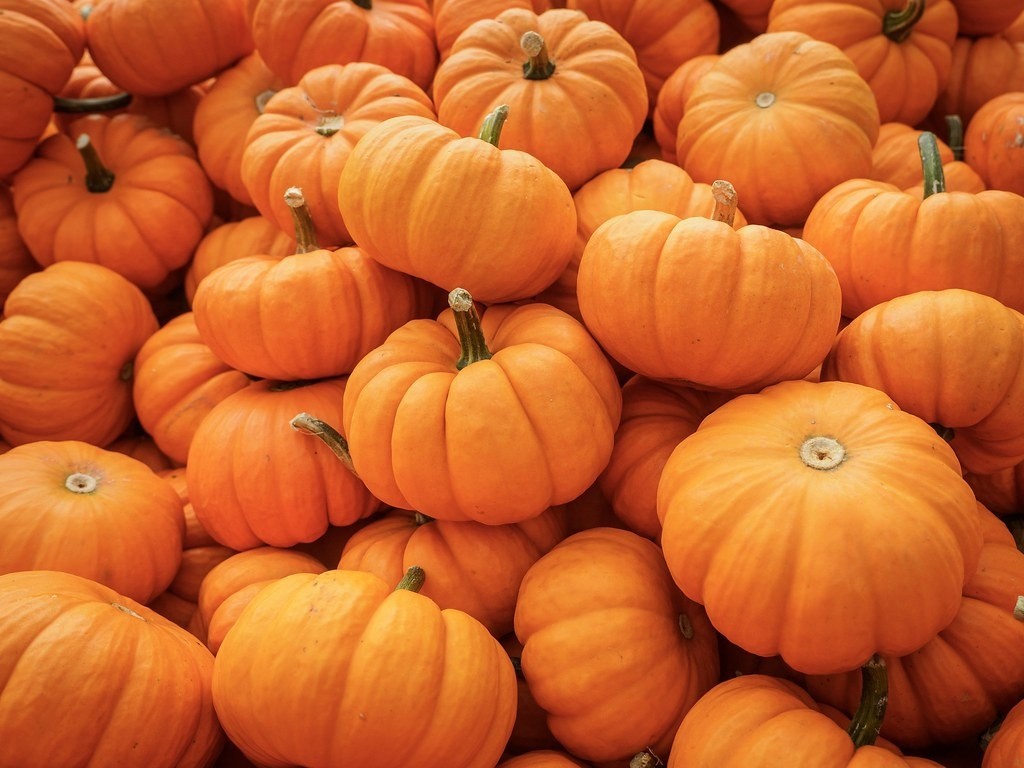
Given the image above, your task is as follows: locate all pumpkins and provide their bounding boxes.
[0,0,1024,768]
